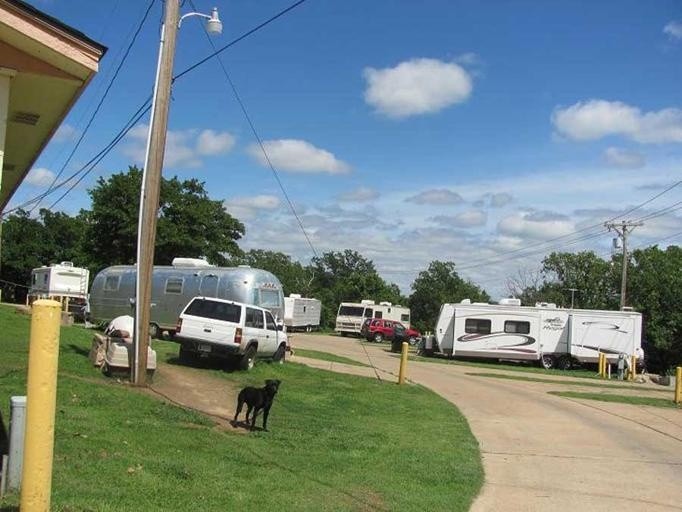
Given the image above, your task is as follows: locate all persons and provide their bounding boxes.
[395,323,405,337]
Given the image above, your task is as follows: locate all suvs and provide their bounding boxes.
[173,295,291,371]
[360,317,422,345]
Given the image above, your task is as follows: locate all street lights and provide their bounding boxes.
[127,5,226,380]
[613,234,628,311]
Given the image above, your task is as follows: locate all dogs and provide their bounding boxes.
[232,379,282,432]
